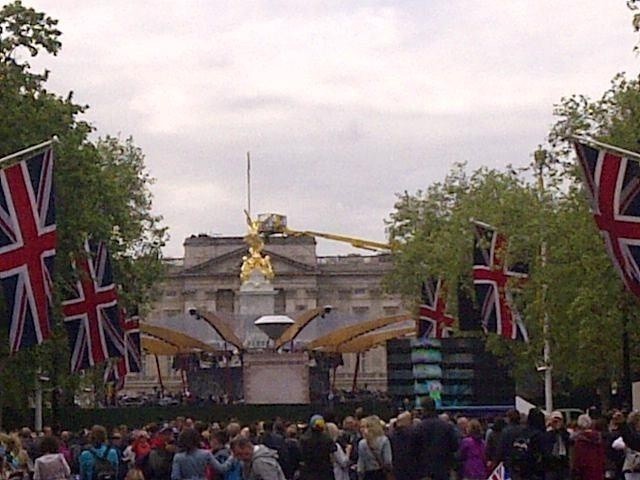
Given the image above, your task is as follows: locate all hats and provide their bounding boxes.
[491,419,506,430]
[414,398,435,411]
[309,415,325,432]
[550,411,563,419]
[578,416,593,427]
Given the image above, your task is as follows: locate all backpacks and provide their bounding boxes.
[509,427,541,479]
[87,444,118,480]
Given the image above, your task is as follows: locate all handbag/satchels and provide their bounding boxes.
[386,472,397,480]
[623,451,640,471]
[224,461,241,480]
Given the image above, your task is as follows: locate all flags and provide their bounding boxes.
[470,221,531,345]
[417,268,457,339]
[110,254,141,374]
[0,144,60,356]
[56,202,125,371]
[570,137,640,299]
